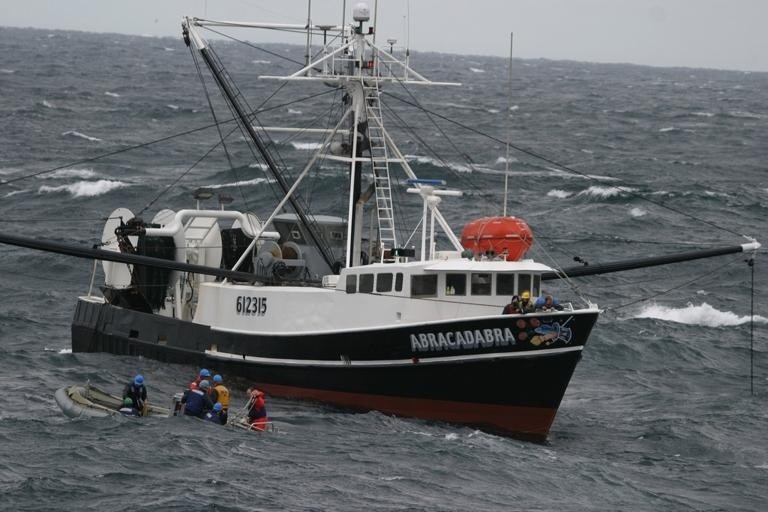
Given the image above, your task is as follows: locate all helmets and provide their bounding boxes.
[134,375,144,385]
[521,292,530,298]
[213,403,222,411]
[199,369,210,377]
[124,398,132,405]
[199,380,209,391]
[213,375,222,382]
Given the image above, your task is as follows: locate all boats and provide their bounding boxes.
[52,384,285,441]
[0,0,760,446]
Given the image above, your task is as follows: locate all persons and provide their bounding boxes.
[519,290,535,315]
[204,402,225,425]
[181,380,212,419]
[535,295,564,312]
[124,374,148,411]
[118,398,138,418]
[211,374,229,421]
[501,295,524,315]
[246,387,267,429]
[190,367,211,397]
